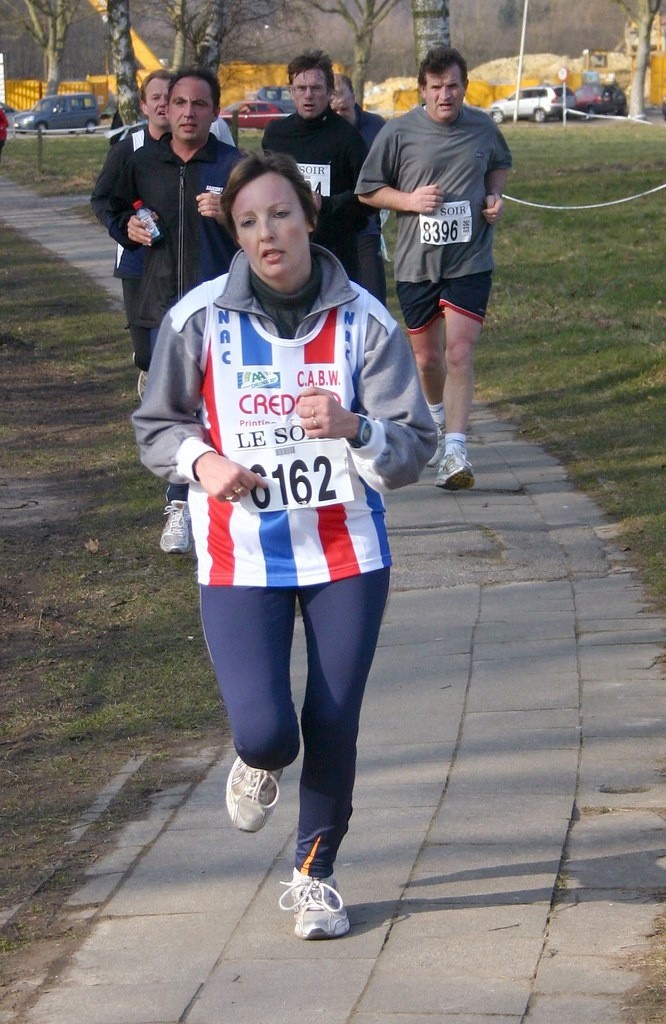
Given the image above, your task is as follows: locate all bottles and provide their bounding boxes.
[133,201,160,239]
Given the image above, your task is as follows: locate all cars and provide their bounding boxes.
[217,100,282,130]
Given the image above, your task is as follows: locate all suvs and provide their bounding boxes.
[574,81,627,118]
[487,83,577,124]
[250,84,296,114]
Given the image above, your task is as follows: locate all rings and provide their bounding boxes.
[312,408,315,416]
[311,417,317,427]
[225,495,235,501]
[234,486,243,493]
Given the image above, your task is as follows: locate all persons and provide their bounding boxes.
[104,69,242,554]
[259,51,368,283]
[350,47,512,494]
[329,74,387,308]
[91,71,175,402]
[131,153,438,940]
[0,108,9,161]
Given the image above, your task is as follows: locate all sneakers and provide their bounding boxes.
[159,500,192,553]
[433,444,475,491]
[426,422,446,467]
[226,756,283,833]
[278,867,350,940]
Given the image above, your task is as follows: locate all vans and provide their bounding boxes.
[12,94,100,136]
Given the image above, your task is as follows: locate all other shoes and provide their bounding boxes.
[137,370,149,401]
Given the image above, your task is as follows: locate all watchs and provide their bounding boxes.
[346,415,372,449]
